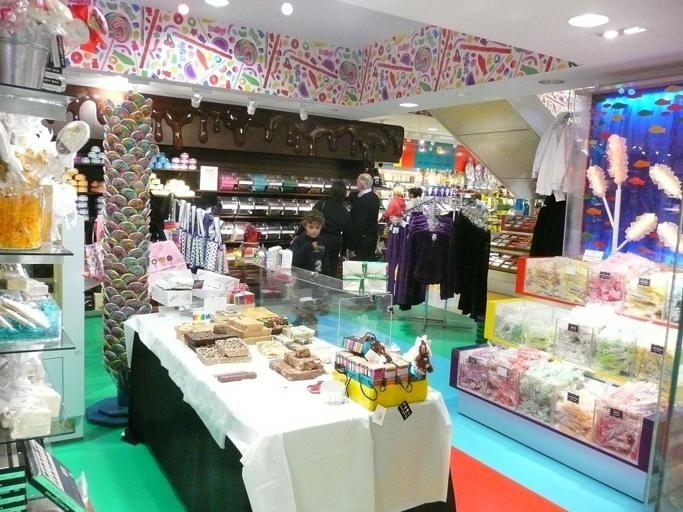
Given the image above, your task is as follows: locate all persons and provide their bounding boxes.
[287,210,325,334]
[311,182,351,310]
[403,187,423,210]
[383,186,405,221]
[350,173,377,261]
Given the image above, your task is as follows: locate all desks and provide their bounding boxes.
[127,313,443,511]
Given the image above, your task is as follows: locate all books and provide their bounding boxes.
[24,438,87,511]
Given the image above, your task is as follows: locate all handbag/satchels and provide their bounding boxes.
[84,241,105,284]
[148,220,229,293]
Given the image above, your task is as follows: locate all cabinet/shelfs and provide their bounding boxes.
[76,140,357,247]
[450,255,682,504]
[0,82,75,512]
